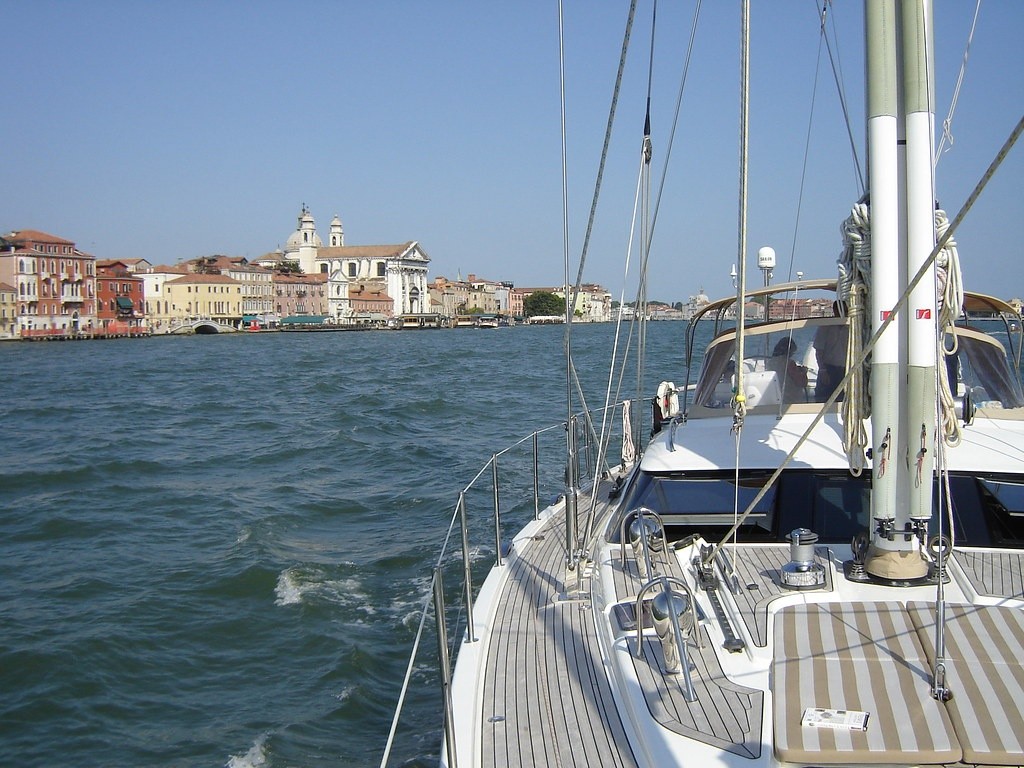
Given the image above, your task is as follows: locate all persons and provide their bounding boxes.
[767,336,808,404]
[812,300,849,404]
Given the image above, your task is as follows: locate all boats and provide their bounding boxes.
[477,315,499,328]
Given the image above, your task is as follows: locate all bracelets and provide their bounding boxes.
[819,369,827,371]
